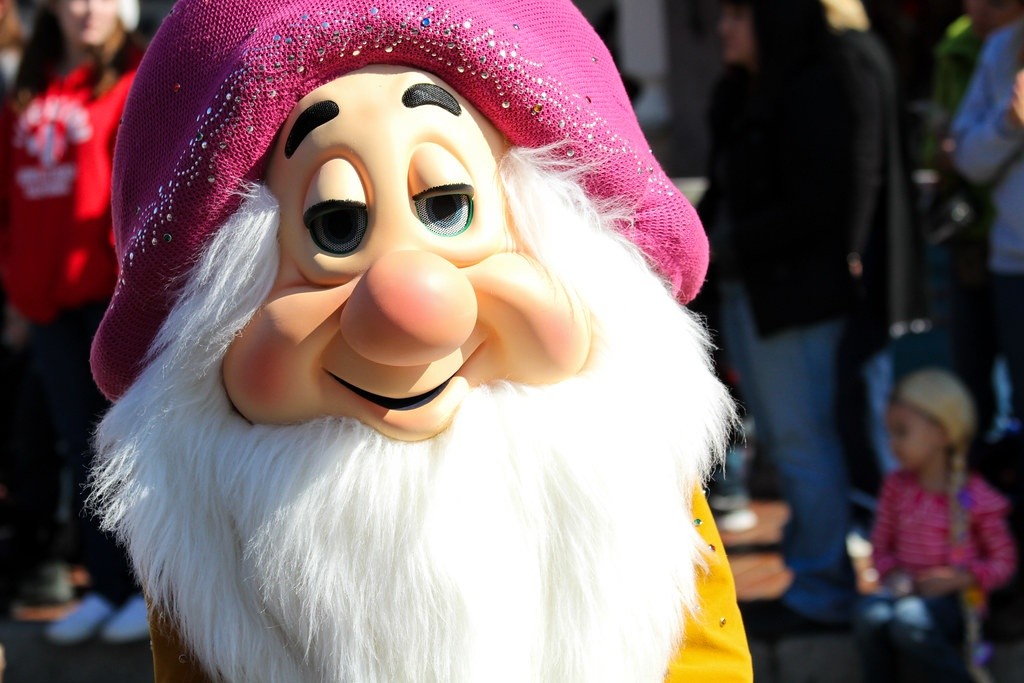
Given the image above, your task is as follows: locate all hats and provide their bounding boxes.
[897,369,974,445]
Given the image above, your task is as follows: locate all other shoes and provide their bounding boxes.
[101,597,153,642]
[745,598,851,642]
[43,592,115,644]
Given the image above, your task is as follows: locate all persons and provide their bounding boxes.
[0,0,151,642]
[694,0,1024,638]
[852,369,1016,683]
[90,1,753,683]
